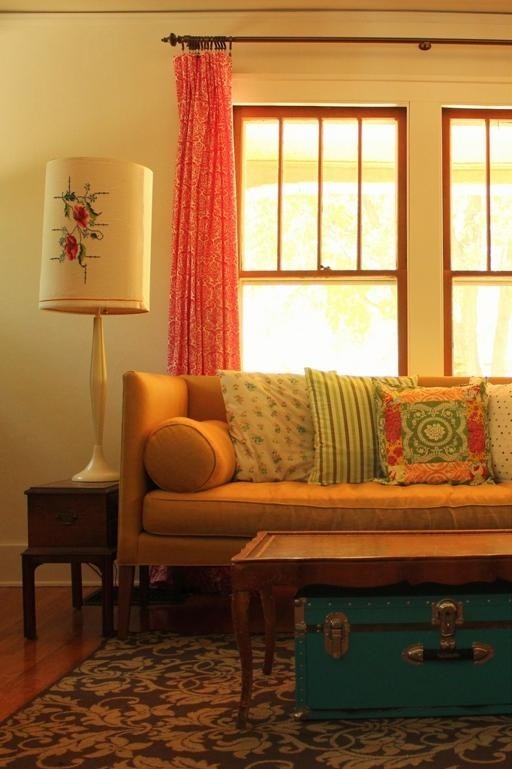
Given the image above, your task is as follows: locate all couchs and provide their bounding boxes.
[115,368,512,639]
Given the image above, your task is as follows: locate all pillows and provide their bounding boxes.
[469,376,512,484]
[370,378,493,487]
[218,370,315,479]
[145,417,236,492]
[306,369,418,485]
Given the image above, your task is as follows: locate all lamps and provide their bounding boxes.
[38,156,154,481]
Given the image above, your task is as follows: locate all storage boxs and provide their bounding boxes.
[24,483,118,546]
[293,581,512,720]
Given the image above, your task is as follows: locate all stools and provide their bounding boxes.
[20,545,117,639]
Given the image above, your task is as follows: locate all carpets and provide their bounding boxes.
[1,626,512,769]
